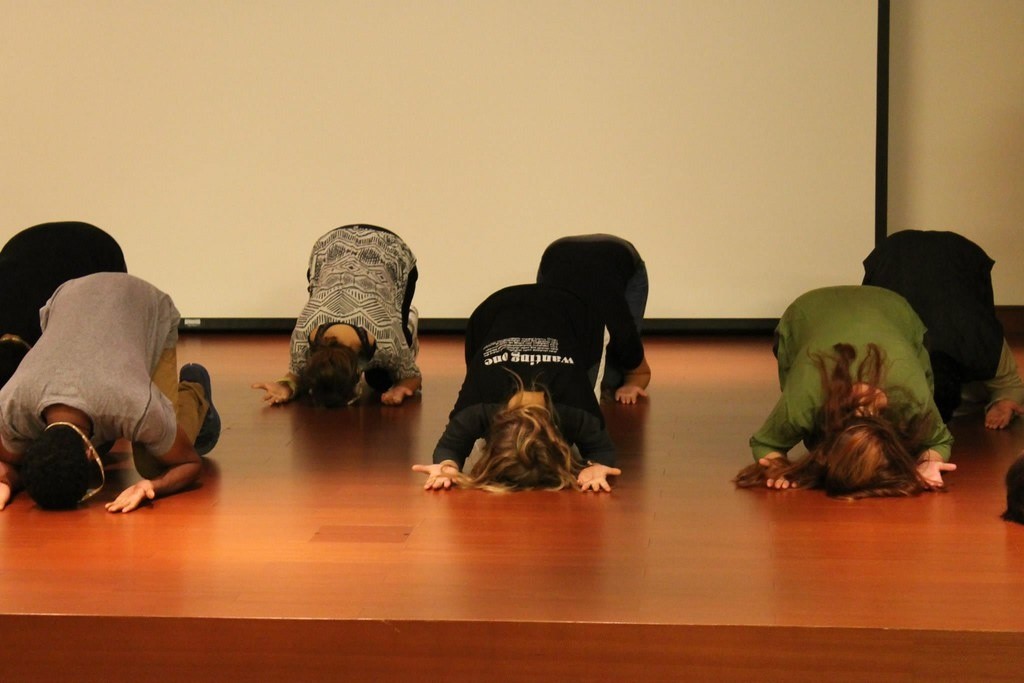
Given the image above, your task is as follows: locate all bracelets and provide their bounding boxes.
[277,377,298,398]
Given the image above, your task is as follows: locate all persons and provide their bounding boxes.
[732,284,957,503]
[0,219,220,513]
[411,234,651,489]
[251,223,422,409]
[862,231,1024,523]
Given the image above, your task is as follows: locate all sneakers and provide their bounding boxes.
[407,305,419,361]
[180,363,221,455]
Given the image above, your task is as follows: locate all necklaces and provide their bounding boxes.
[72,423,91,434]
